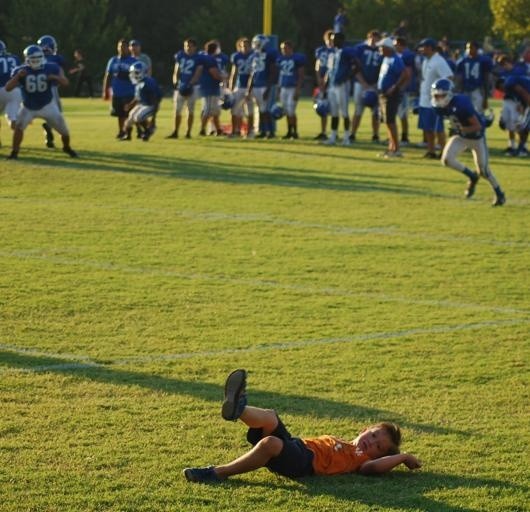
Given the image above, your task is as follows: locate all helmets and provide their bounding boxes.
[430,78,456,109]
[271,105,284,119]
[251,34,269,53]
[315,100,330,117]
[22,45,47,70]
[37,35,57,55]
[128,61,148,85]
[362,90,378,108]
[218,92,235,110]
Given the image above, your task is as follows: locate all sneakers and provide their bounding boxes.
[465,173,480,196]
[493,192,505,207]
[63,146,79,157]
[182,466,228,484]
[222,369,247,420]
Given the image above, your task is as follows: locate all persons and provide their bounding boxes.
[102,37,160,140]
[184,367,423,484]
[165,33,306,140]
[312,9,530,206]
[0,36,93,158]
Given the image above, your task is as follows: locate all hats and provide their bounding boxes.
[375,38,394,49]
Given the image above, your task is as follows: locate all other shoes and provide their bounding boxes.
[312,134,409,158]
[425,153,436,159]
[434,144,441,149]
[517,146,529,158]
[416,142,427,148]
[504,148,516,156]
[116,124,156,141]
[166,129,298,140]
